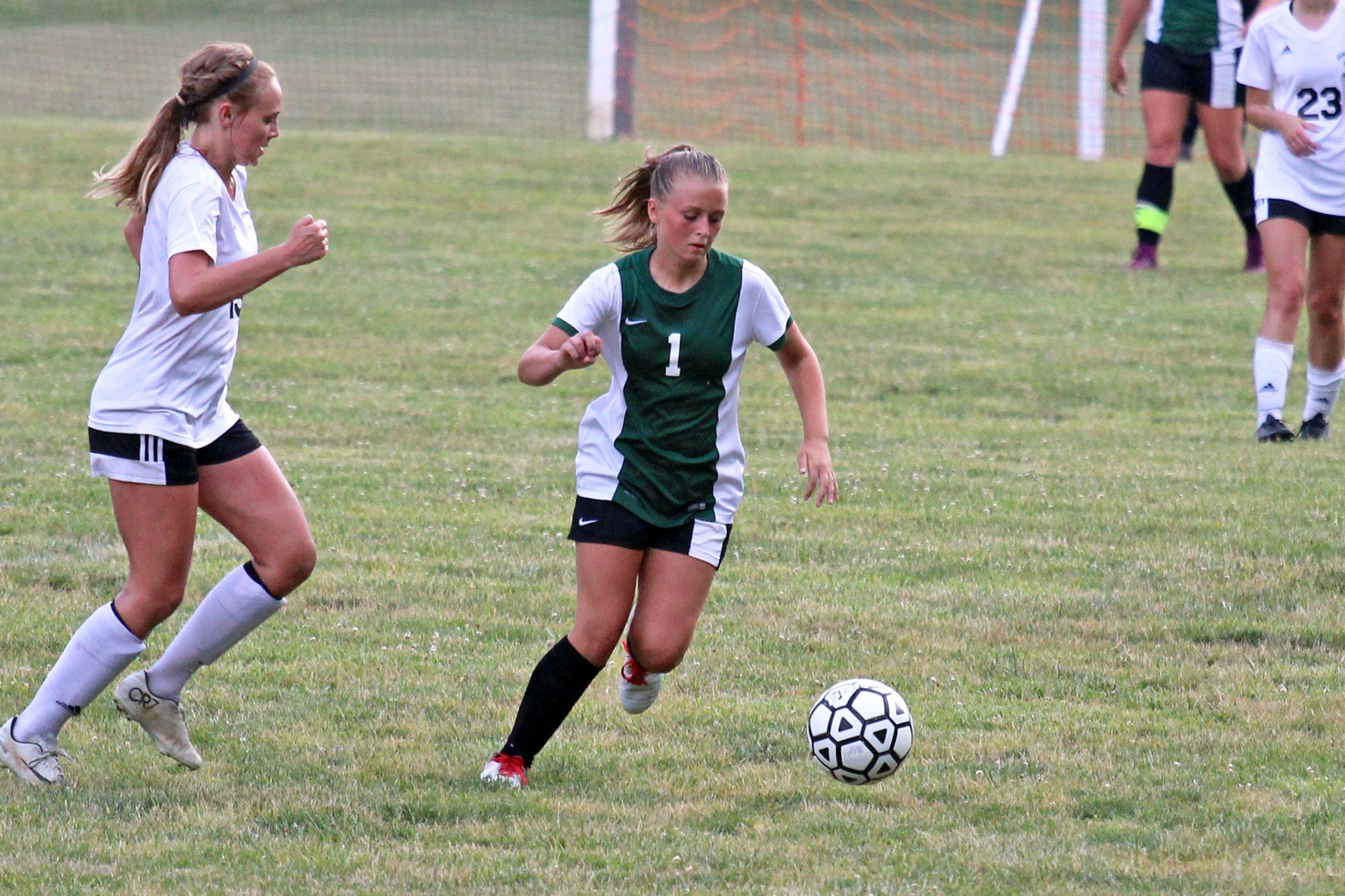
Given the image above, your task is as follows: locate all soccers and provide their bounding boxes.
[806,678,915,786]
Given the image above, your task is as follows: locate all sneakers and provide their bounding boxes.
[113,670,203,770]
[620,639,664,715]
[480,752,527,793]
[1241,231,1266,272]
[1122,245,1157,272]
[1255,414,1294,443]
[1298,413,1331,439]
[0,718,79,795]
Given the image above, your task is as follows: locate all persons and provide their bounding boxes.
[481,144,839,786]
[0,42,329,794]
[1234,0,1345,442]
[1112,0,1263,271]
